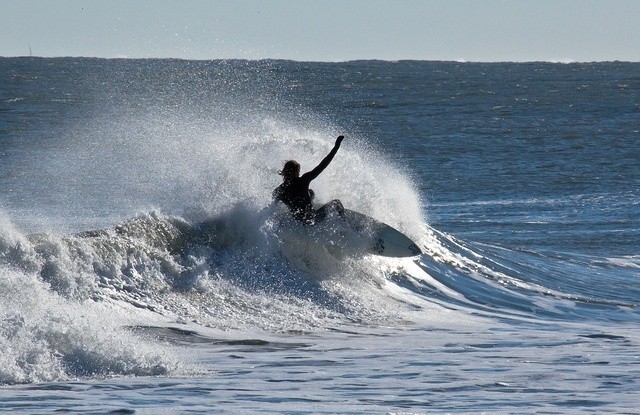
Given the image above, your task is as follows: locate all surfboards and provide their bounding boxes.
[315,203,423,257]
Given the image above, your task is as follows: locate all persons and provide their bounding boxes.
[273,136,344,227]
